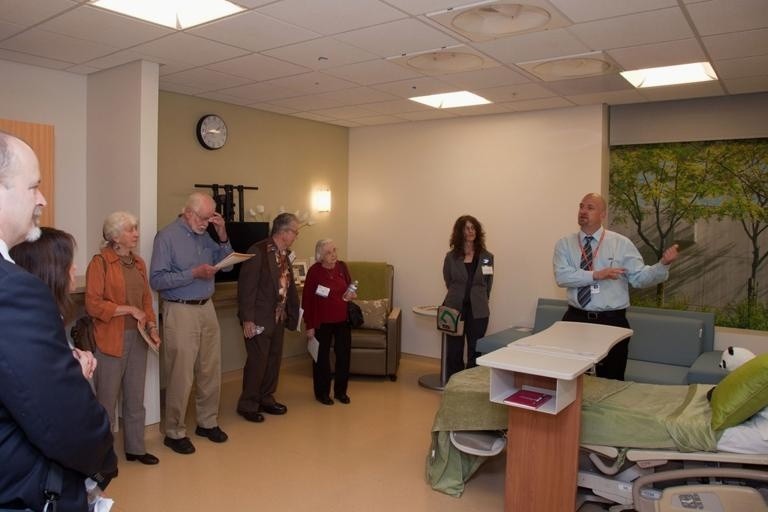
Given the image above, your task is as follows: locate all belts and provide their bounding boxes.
[167,299,210,305]
[569,307,624,320]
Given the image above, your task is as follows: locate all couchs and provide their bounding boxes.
[471,297,734,389]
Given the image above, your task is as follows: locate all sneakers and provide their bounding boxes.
[163,435,195,454]
[195,425,228,443]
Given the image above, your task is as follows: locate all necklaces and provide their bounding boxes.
[117,253,136,269]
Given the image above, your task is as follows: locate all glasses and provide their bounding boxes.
[193,210,214,221]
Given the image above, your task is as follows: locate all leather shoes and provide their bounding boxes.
[236,406,264,422]
[334,391,350,404]
[259,401,287,414]
[315,394,334,406]
[125,452,159,464]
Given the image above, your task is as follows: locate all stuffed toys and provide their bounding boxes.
[705,347,758,402]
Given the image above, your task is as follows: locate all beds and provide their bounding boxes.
[422,345,768,511]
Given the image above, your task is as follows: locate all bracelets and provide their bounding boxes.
[219,239,230,244]
[148,324,157,331]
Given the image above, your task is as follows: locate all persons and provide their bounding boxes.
[148,190,237,456]
[0,130,120,511]
[302,237,358,406]
[9,226,99,381]
[234,211,302,424]
[552,191,680,381]
[443,215,496,384]
[83,210,162,466]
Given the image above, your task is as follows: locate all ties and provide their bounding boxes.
[577,236,593,308]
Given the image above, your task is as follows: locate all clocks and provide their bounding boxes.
[196,113,230,151]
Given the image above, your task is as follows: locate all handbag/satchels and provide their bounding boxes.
[348,301,364,328]
[436,306,464,337]
[71,316,98,355]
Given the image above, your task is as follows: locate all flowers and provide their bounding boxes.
[247,204,318,233]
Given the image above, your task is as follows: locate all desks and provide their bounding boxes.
[474,318,638,512]
[412,302,465,393]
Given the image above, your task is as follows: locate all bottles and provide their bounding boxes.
[255,325,265,336]
[342,279,359,301]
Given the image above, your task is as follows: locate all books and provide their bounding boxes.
[136,319,159,354]
[213,250,257,272]
[501,388,551,410]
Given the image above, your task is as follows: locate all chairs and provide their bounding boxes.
[304,260,402,381]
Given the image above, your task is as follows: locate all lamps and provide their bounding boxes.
[317,184,332,214]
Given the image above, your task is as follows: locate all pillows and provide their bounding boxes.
[348,297,390,332]
[707,349,767,433]
